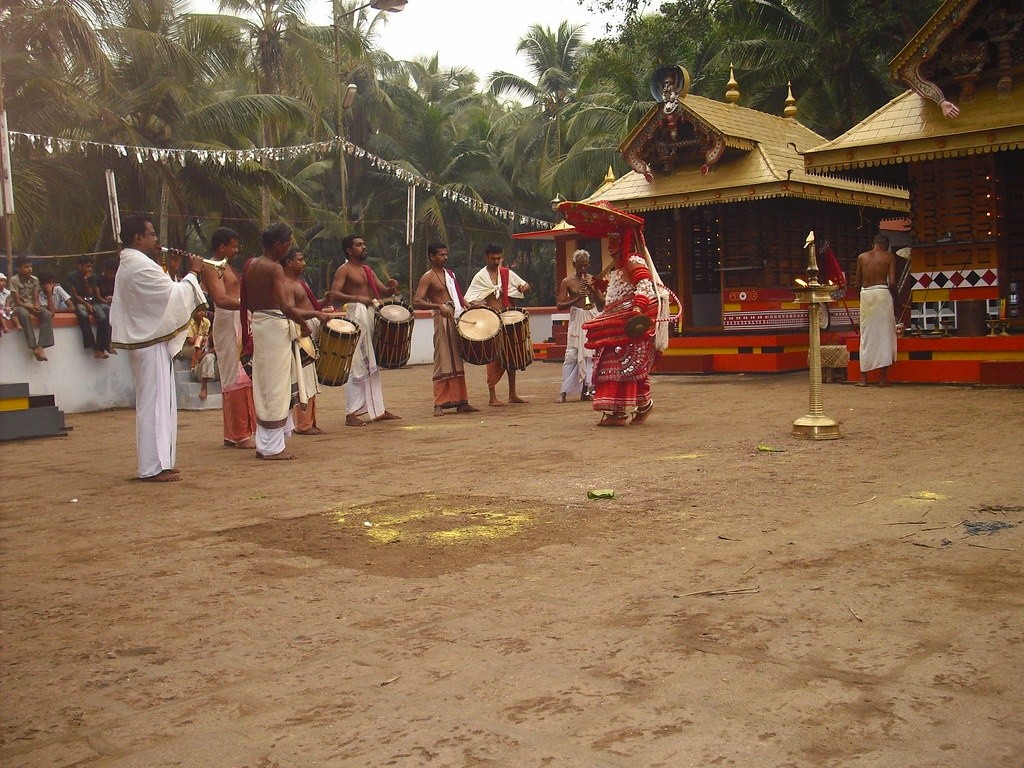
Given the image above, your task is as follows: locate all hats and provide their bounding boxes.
[0,273,7,279]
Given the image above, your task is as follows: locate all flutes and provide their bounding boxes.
[582,273,595,310]
[156,245,228,280]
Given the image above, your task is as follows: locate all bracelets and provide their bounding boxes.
[190,271,198,278]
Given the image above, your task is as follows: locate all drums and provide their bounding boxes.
[373,300,415,369]
[240,323,320,381]
[456,306,503,366]
[316,316,361,387]
[498,307,533,371]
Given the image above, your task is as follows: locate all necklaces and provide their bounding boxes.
[432,267,446,286]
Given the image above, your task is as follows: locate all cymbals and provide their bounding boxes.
[624,314,652,338]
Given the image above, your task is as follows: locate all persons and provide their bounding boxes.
[240,223,312,460]
[557,249,606,403]
[0,273,23,332]
[855,235,897,387]
[95,264,118,355]
[110,215,253,482]
[282,248,332,435]
[332,236,398,426]
[464,243,531,406]
[68,256,110,359]
[413,243,481,416]
[9,254,55,361]
[558,233,682,426]
[38,273,75,317]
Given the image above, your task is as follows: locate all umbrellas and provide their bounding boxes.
[824,242,860,336]
